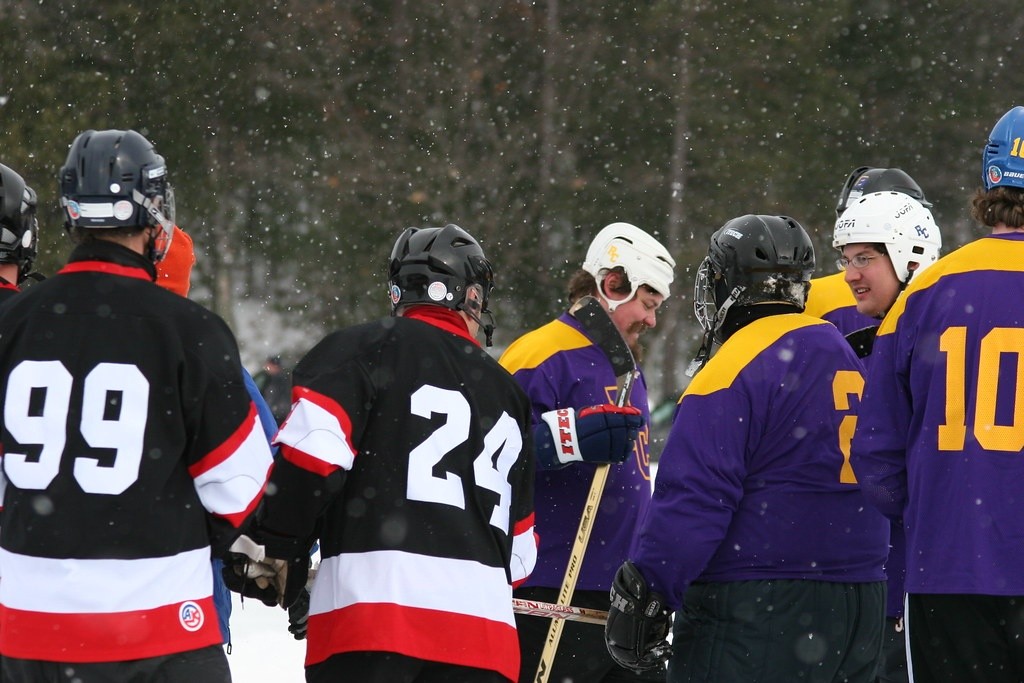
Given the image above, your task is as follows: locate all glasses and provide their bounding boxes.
[834,248,887,272]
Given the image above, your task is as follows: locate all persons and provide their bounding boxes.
[270,223,541,683]
[0,128,317,683]
[255,355,280,394]
[600,166,971,683]
[851,103,1024,683]
[499,220,667,683]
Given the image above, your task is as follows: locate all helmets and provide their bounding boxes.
[692,212,816,345]
[836,166,933,209]
[57,130,175,265]
[832,191,943,285]
[386,223,496,312]
[980,105,1024,191]
[582,221,677,312]
[0,162,39,273]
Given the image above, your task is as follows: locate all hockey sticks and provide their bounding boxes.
[239,558,674,633]
[531,294,638,683]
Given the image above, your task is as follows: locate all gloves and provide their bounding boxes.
[539,402,647,467]
[221,526,302,607]
[289,586,311,639]
[604,559,674,673]
